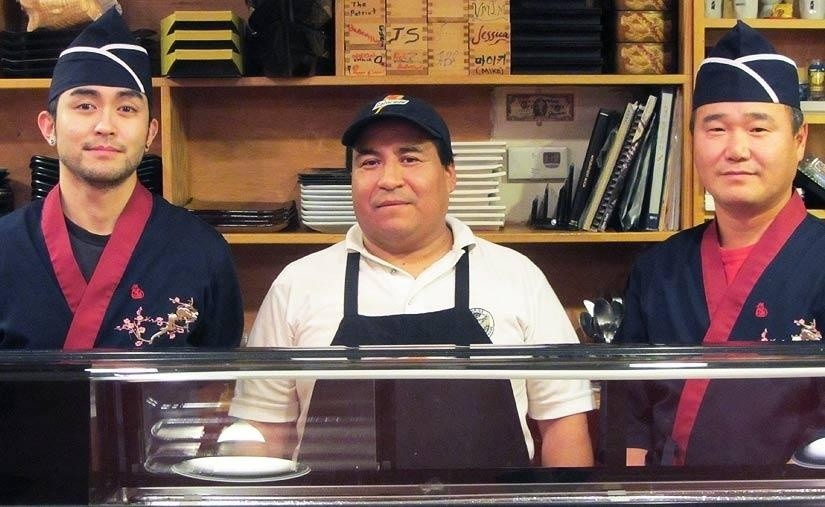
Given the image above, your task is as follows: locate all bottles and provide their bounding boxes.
[809,58,824,101]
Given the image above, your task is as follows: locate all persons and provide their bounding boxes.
[212,95,592,469]
[598,21,823,470]
[0,5,244,507]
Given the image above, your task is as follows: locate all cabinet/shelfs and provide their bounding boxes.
[0,1,825,243]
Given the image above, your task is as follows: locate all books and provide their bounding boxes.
[561,91,683,233]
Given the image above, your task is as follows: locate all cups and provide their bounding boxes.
[704,0,825,20]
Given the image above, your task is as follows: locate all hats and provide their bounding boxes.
[691,21,800,111]
[341,93,450,148]
[49,4,152,104]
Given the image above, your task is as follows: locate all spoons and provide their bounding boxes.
[580,296,625,343]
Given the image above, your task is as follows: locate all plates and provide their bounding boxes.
[0,28,160,77]
[30,155,163,204]
[170,455,312,483]
[183,200,296,233]
[446,142,509,231]
[0,168,19,217]
[297,167,357,233]
